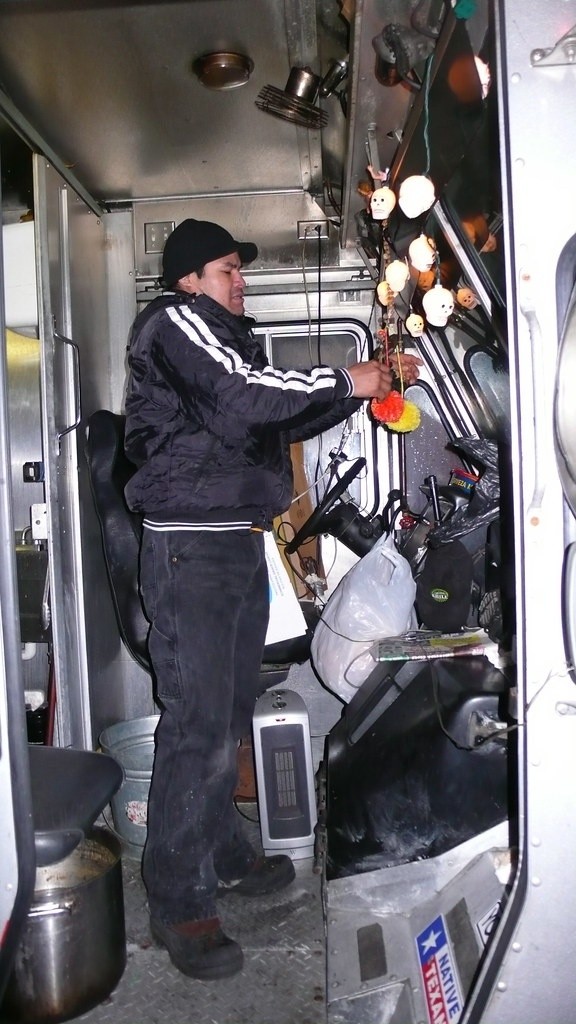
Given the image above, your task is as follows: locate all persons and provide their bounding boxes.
[122,220,425,979]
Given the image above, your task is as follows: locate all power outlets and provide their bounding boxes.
[144,221,176,254]
[295,221,331,240]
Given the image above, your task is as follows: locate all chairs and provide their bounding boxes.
[84,410,324,698]
[26,745,126,866]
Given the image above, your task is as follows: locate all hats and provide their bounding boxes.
[415,541,474,633]
[163,219,258,280]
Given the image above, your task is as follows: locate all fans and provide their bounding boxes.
[253,51,352,128]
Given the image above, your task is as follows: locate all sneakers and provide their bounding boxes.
[150,912,243,980]
[215,853,295,898]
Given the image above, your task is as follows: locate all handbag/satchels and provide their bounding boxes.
[310,529,419,705]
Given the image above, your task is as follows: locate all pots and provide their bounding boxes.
[6,822,125,1023]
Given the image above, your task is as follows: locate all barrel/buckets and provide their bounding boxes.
[99,714,160,845]
